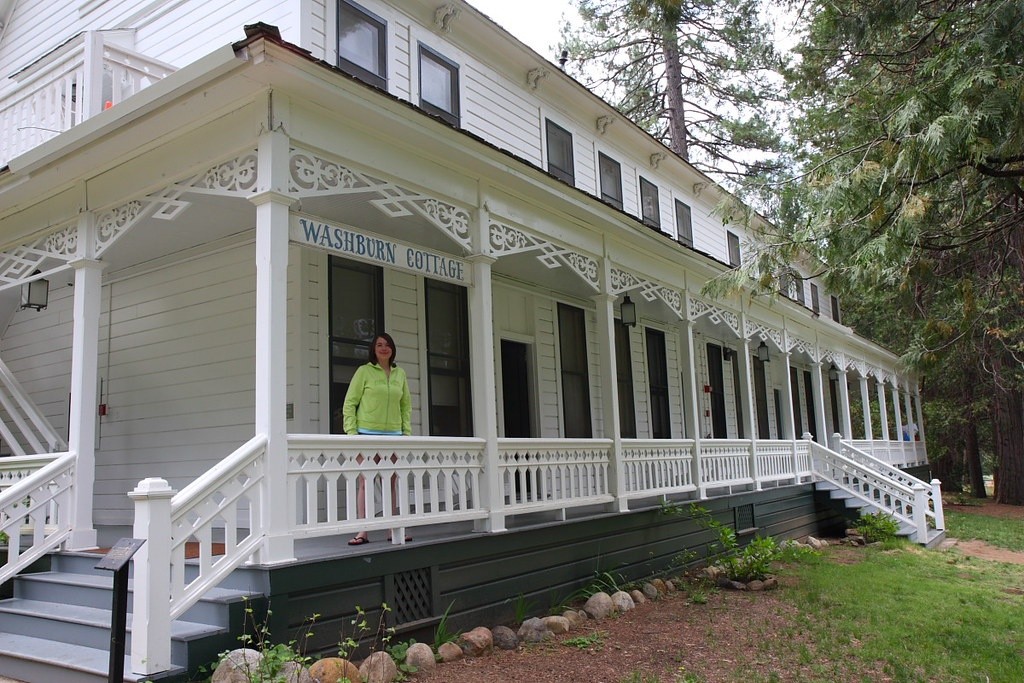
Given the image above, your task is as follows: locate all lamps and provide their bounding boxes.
[829,363,837,381]
[21,269,49,312]
[758,340,770,361]
[620,290,636,327]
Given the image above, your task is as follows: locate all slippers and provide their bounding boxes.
[348,537,369,545]
[387,534,412,542]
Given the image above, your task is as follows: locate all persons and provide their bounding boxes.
[341,332,413,545]
